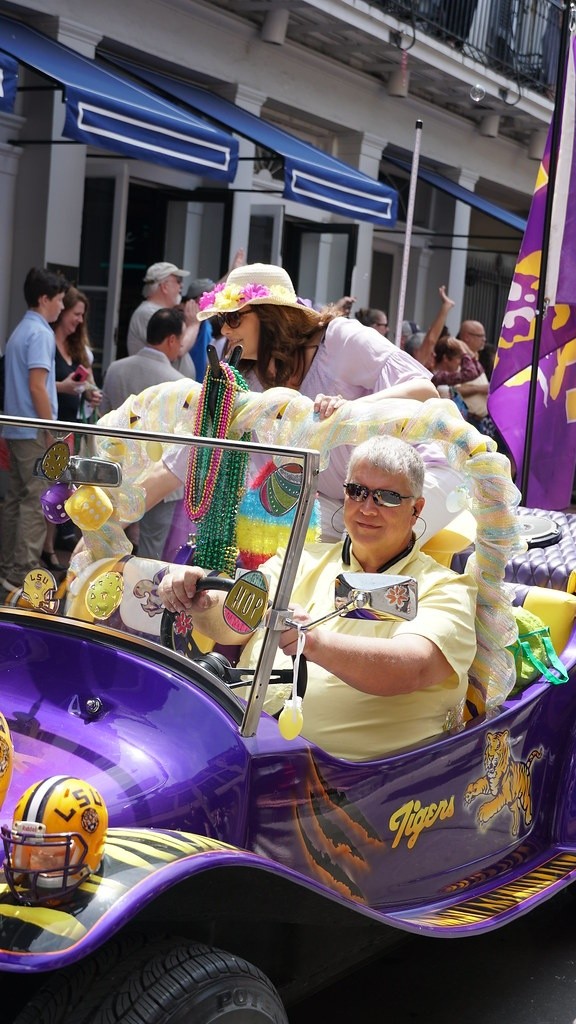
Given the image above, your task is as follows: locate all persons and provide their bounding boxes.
[156,438,475,762]
[2,267,70,591]
[127,262,201,383]
[49,285,102,571]
[187,248,244,382]
[99,308,186,563]
[355,287,502,442]
[101,260,473,553]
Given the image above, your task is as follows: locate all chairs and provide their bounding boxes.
[76,553,576,725]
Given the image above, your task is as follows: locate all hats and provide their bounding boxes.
[143,261,191,283]
[196,262,322,322]
[188,280,215,301]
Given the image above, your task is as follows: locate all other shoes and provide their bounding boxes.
[40,548,69,571]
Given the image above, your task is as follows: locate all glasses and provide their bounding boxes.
[216,310,257,328]
[343,481,416,507]
[373,322,389,328]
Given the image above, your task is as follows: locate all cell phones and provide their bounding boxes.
[72,365,88,383]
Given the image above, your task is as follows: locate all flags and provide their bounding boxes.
[487,28,576,511]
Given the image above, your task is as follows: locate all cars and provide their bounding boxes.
[1,416,576,1024]
